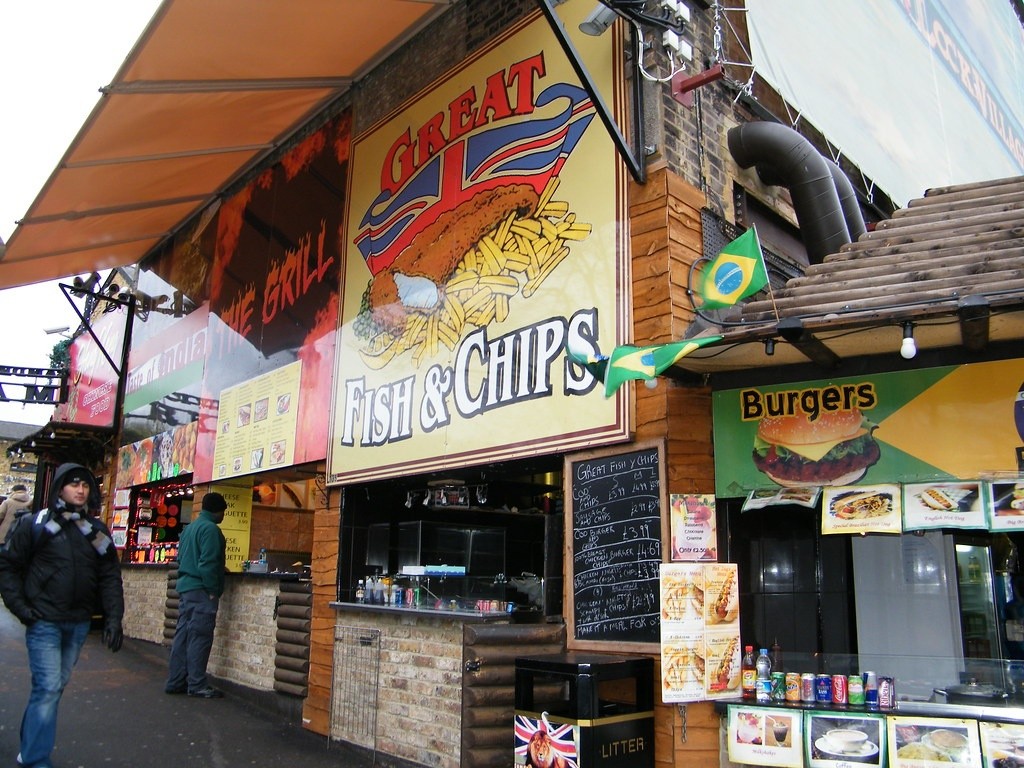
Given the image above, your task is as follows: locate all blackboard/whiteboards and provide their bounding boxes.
[563,438,670,654]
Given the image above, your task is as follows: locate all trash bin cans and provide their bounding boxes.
[513,651,655,768]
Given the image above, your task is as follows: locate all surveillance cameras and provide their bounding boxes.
[103,283,119,296]
[43,325,70,334]
[117,286,130,302]
[578,3,619,37]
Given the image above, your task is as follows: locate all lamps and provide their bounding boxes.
[166,487,194,497]
[422,489,432,507]
[457,486,469,504]
[475,486,488,503]
[440,487,451,505]
[405,492,414,508]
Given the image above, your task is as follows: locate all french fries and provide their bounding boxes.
[899,743,950,761]
[662,574,690,620]
[662,642,689,690]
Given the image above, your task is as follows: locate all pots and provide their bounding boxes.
[933,677,1015,708]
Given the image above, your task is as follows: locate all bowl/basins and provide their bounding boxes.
[826,729,868,752]
[249,563,268,573]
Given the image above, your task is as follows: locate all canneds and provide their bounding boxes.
[861,670,895,708]
[477,600,516,614]
[391,584,421,606]
[771,671,865,705]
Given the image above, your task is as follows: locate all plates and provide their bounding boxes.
[895,730,971,763]
[987,723,1024,768]
[814,736,879,757]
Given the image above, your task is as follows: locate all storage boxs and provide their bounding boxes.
[242,563,269,572]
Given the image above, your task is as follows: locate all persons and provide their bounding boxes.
[165,493,227,699]
[0,485,33,546]
[0,463,124,768]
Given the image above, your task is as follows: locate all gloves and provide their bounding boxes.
[101,609,123,652]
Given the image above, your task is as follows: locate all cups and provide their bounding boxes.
[772,725,789,747]
[738,721,758,742]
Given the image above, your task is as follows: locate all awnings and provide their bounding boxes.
[0,0,458,292]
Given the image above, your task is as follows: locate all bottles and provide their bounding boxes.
[741,645,757,699]
[756,649,773,701]
[258,548,267,564]
[966,555,982,583]
[356,577,399,604]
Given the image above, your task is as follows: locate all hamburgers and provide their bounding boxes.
[751,401,880,488]
[930,731,967,752]
[691,641,705,685]
[691,568,704,615]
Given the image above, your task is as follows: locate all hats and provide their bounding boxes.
[61,473,87,489]
[202,493,228,513]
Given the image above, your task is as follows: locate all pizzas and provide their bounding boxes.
[118,439,154,485]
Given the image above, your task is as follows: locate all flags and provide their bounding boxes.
[691,226,770,312]
[563,335,725,398]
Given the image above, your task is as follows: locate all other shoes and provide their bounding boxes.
[16,752,22,765]
[188,687,225,698]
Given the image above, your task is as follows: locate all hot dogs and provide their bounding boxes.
[712,567,738,622]
[833,491,886,517]
[921,488,954,510]
[716,636,741,690]
